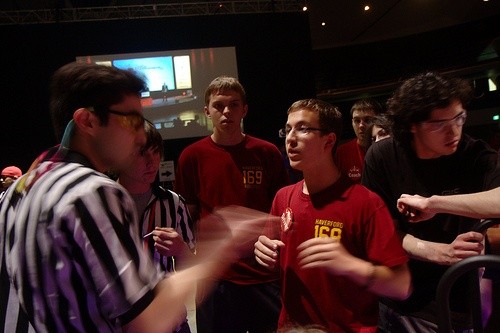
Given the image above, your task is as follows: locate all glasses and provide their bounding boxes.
[107,108,145,133]
[278,125,322,138]
[423,111,466,132]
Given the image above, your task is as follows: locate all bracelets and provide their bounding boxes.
[363,262,376,289]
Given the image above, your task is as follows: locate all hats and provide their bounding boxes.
[1,166,23,178]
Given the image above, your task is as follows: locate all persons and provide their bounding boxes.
[336,99,386,190]
[175,74,290,333]
[361,72,500,333]
[254,97,412,333]
[0,63,281,332]
